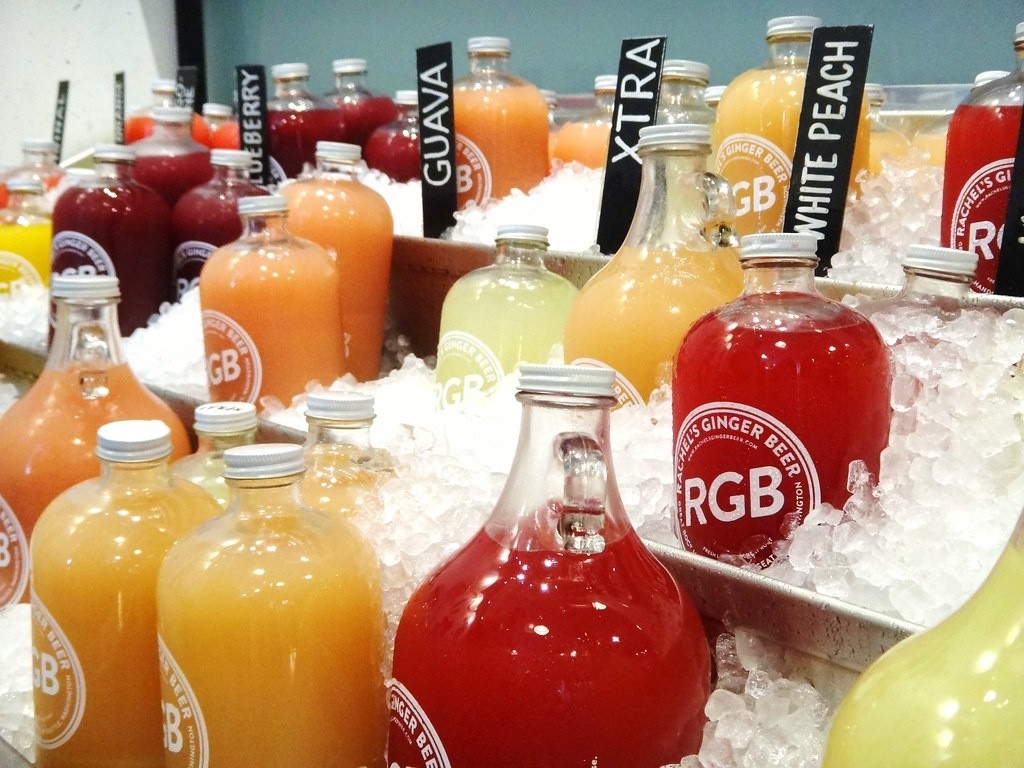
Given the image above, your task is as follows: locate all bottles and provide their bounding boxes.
[0,57,424,362]
[286,389,399,530]
[821,498,1021,768]
[668,233,894,566]
[709,13,872,245]
[938,17,1024,298]
[29,417,225,768]
[196,193,347,421]
[555,72,622,170]
[859,79,911,177]
[561,122,784,420]
[434,223,580,413]
[449,34,552,214]
[0,270,189,614]
[165,402,259,514]
[654,60,718,149]
[265,141,394,384]
[155,443,388,768]
[846,241,999,411]
[379,359,716,767]
